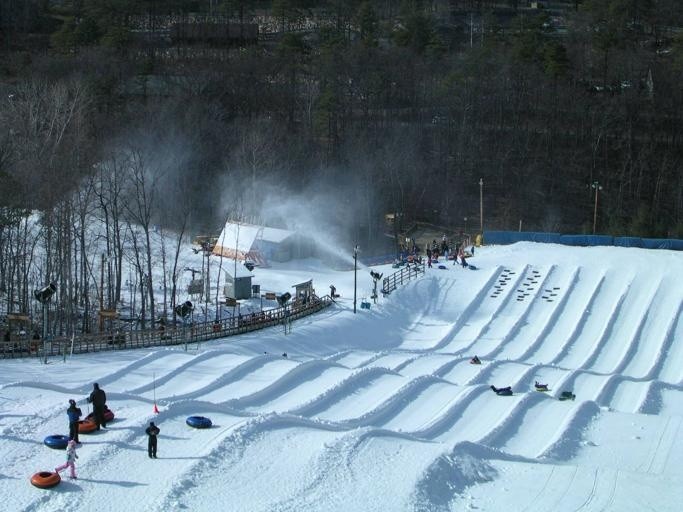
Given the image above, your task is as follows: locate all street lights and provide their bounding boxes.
[351,240,361,314]
[478,178,484,234]
[591,182,603,234]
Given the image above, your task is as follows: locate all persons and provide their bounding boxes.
[145,422,160,459]
[66,399,82,444]
[397,234,475,269]
[472,354,479,363]
[535,381,548,388]
[86,383,106,431]
[302,296,310,309]
[329,284,336,300]
[3,309,265,353]
[54,437,79,479]
[489,385,512,393]
